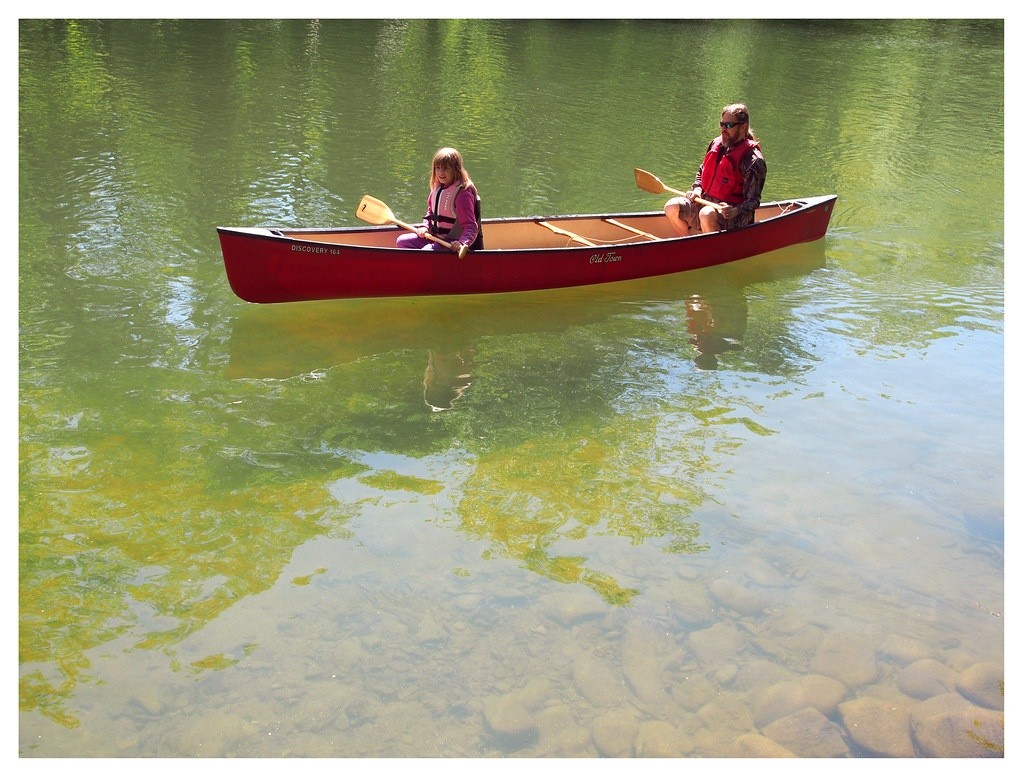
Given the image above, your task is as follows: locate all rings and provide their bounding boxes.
[727,214,728,216]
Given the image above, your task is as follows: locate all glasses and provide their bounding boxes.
[719,122,739,128]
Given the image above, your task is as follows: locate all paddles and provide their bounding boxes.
[355,194,468,260]
[634,168,727,214]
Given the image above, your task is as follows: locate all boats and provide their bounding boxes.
[216,190,839,303]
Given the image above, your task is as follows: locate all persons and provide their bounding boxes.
[395,147,479,251]
[663,104,768,237]
